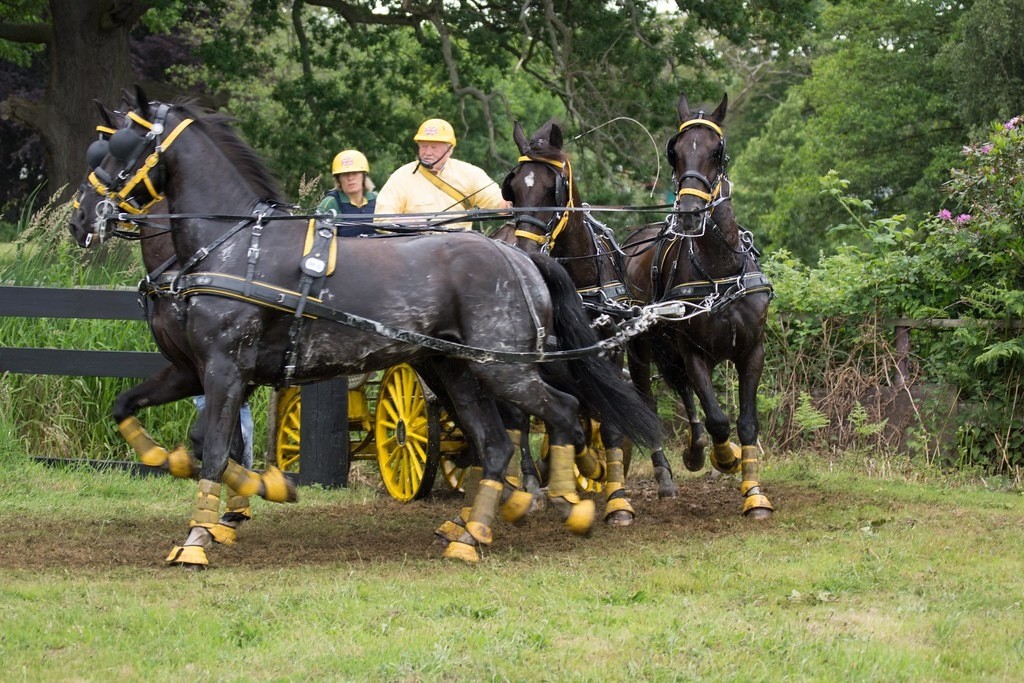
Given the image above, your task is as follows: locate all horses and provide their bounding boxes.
[66,84,666,572]
[624,92,774,522]
[492,120,650,503]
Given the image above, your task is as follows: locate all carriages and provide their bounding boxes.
[64,82,776,572]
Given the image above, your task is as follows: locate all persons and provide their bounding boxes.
[317,149,378,239]
[194,395,254,468]
[373,119,513,235]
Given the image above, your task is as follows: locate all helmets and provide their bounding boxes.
[332,149,370,176]
[414,118,456,148]
